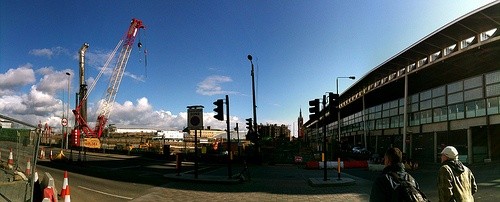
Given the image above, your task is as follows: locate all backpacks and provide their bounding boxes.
[386,170,428,202]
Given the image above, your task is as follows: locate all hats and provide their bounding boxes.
[438,146,458,160]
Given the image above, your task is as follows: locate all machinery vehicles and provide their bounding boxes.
[74,18,147,151]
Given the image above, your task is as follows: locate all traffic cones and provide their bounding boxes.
[25,158,30,174]
[7,148,14,164]
[63,185,71,202]
[59,171,69,196]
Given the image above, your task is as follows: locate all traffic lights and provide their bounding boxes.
[213,99,223,121]
[329,93,340,116]
[309,99,319,120]
[246,118,252,129]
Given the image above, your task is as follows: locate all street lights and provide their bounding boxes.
[247,55,258,156]
[65,72,70,148]
[337,75,355,143]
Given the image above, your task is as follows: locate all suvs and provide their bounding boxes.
[353,147,371,155]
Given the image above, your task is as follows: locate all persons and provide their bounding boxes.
[438,146,477,202]
[369,148,419,202]
